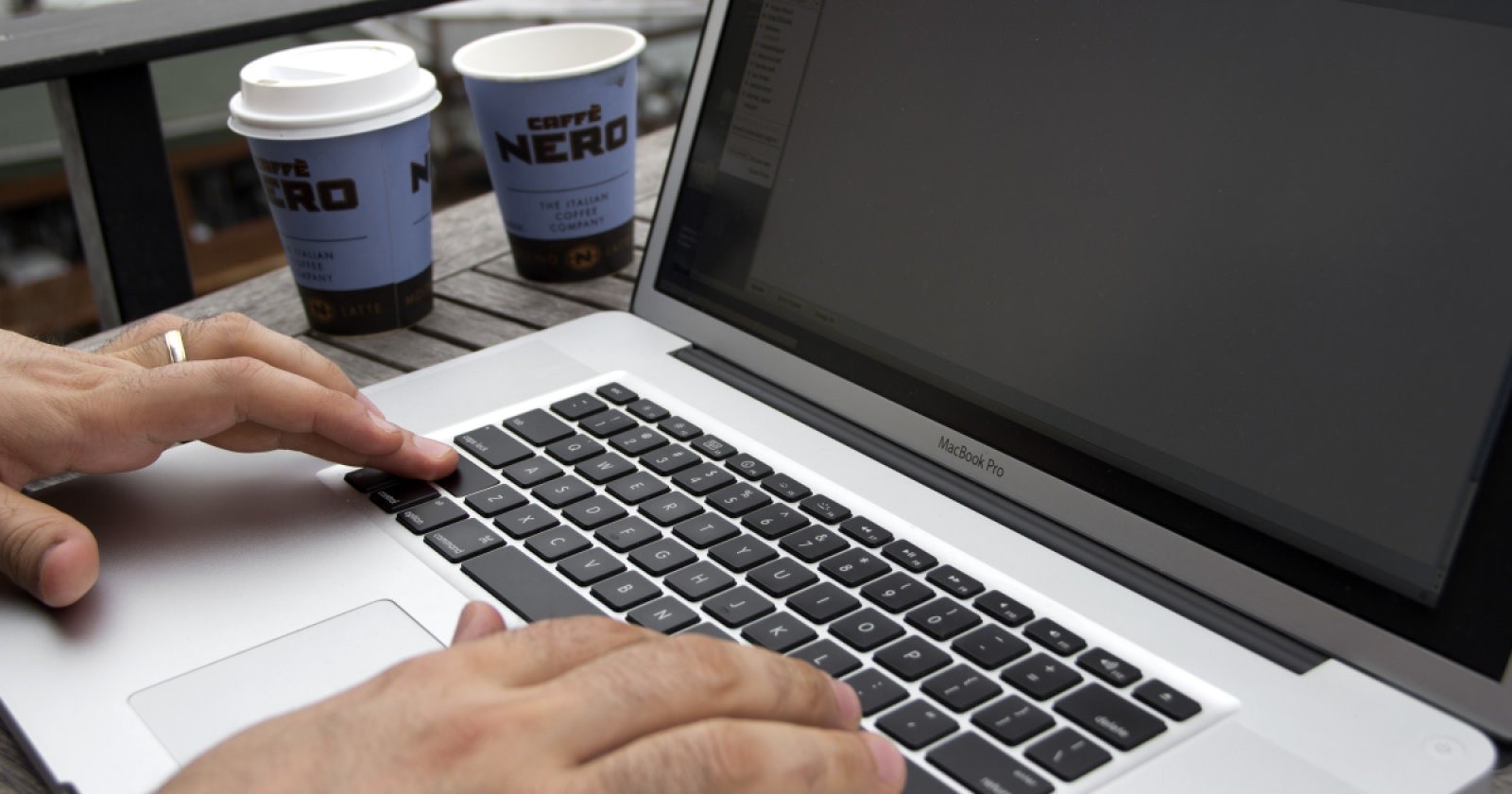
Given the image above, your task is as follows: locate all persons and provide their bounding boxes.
[1,309,918,794]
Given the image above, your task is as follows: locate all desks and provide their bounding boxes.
[0,107,1512,794]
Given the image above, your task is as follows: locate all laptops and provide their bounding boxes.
[3,0,1512,793]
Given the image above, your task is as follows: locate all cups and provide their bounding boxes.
[453,21,649,284]
[227,38,444,336]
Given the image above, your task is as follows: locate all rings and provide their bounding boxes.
[161,328,187,365]
